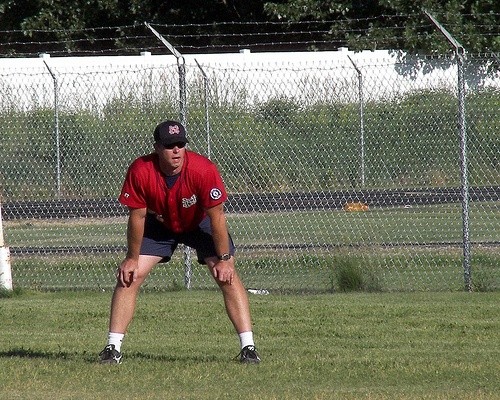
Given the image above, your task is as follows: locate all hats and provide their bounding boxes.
[153,121,188,142]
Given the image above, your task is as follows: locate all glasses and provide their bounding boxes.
[156,142,188,148]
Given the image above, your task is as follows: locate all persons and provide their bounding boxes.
[97,121,262,364]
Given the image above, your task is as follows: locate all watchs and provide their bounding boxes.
[216,253,232,261]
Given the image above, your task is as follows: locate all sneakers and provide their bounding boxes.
[240,346,261,363]
[100,344,123,364]
